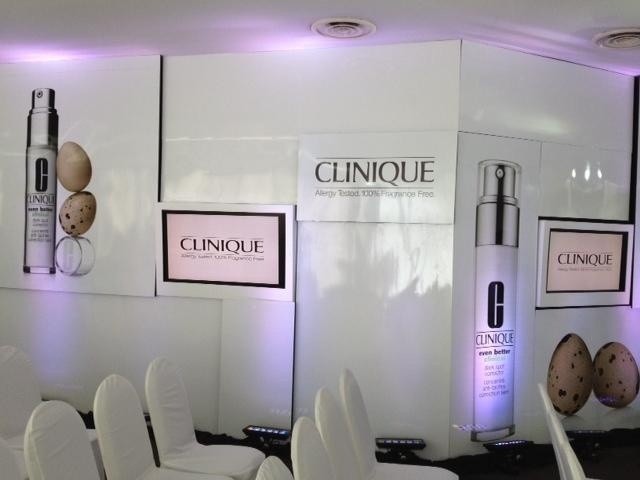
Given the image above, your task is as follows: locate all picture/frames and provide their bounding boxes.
[153,203,296,303]
[536,219,635,308]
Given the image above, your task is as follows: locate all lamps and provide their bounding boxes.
[594,28,639,51]
[312,17,375,39]
[483,440,533,473]
[242,425,291,460]
[568,430,606,462]
[374,438,430,465]
[291,417,334,480]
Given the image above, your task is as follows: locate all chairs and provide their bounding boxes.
[93,374,233,480]
[313,386,362,479]
[340,370,459,480]
[0,345,106,480]
[0,441,26,479]
[144,356,267,480]
[538,382,590,480]
[255,455,293,480]
[25,399,100,480]
